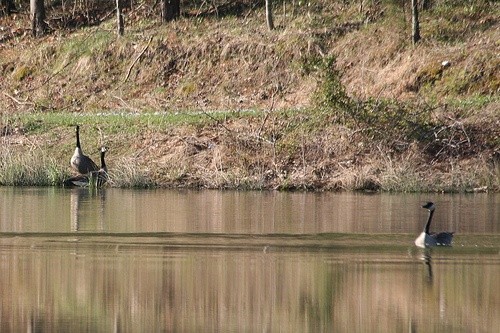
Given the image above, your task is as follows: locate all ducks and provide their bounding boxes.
[62,145,111,189]
[414,199,457,250]
[70,122,100,175]
